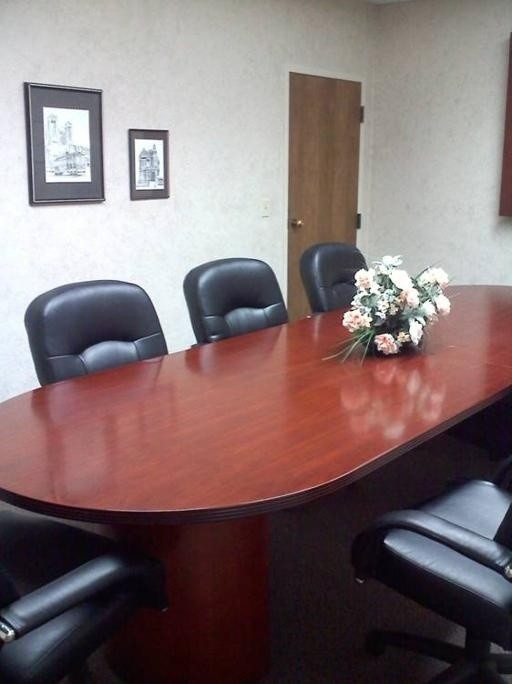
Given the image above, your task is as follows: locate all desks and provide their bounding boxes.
[0,281,511,680]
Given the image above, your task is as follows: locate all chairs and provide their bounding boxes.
[183,257,291,345]
[345,416,512,681]
[298,240,371,317]
[24,280,168,388]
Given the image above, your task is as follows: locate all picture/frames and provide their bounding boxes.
[23,81,106,204]
[128,128,171,201]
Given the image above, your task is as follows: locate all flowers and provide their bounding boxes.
[328,251,452,362]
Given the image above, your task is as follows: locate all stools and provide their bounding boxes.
[0,503,163,681]
